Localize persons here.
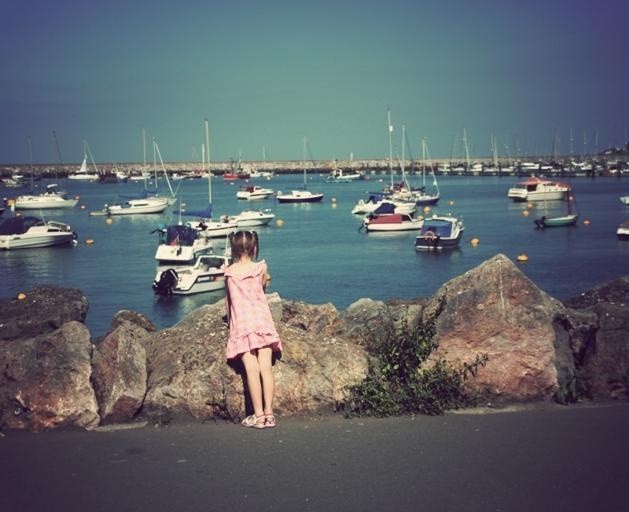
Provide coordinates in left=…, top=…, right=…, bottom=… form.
left=221, top=230, right=284, bottom=428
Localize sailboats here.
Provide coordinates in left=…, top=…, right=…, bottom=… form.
left=2, top=118, right=178, bottom=216
left=328, top=107, right=440, bottom=204
left=150, top=116, right=324, bottom=243
left=438, top=128, right=628, bottom=177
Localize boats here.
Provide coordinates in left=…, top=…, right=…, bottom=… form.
left=352, top=194, right=466, bottom=250
left=0, top=220, right=77, bottom=250
left=154, top=225, right=235, bottom=296
left=535, top=213, right=580, bottom=227
left=616, top=220, right=629, bottom=236
left=507, top=174, right=571, bottom=202
left=620, top=196, right=629, bottom=204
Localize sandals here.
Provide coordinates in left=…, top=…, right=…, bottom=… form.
left=241, top=414, right=276, bottom=429
